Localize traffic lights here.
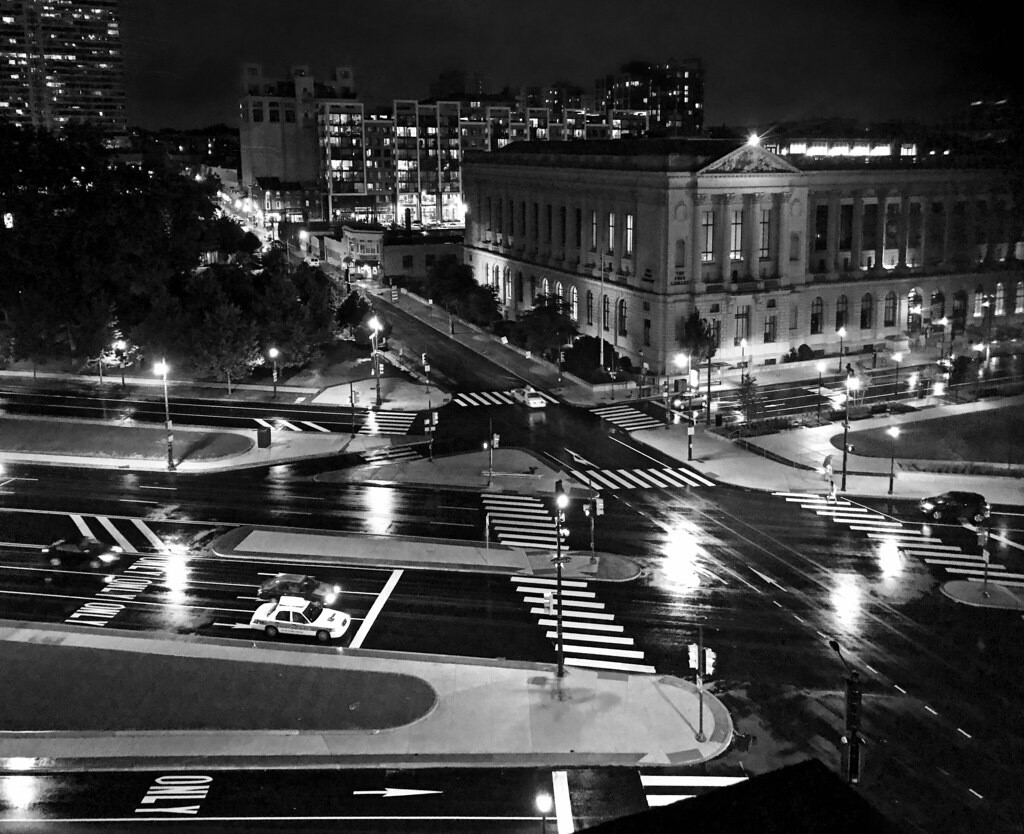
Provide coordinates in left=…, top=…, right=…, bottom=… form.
left=845, top=443, right=854, bottom=453
left=704, top=648, right=718, bottom=676
left=687, top=643, right=700, bottom=672
left=592, top=496, right=605, bottom=518
left=493, top=433, right=500, bottom=448
left=560, top=351, right=565, bottom=363
left=432, top=411, right=438, bottom=428
left=422, top=353, right=427, bottom=367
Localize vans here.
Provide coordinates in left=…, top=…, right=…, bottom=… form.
left=303, top=255, right=320, bottom=267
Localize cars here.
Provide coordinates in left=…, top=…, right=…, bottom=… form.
left=668, top=389, right=708, bottom=410
left=368, top=315, right=384, bottom=405
left=41, top=536, right=125, bottom=570
left=249, top=595, right=351, bottom=644
left=510, top=384, right=547, bottom=408
left=257, top=573, right=343, bottom=609
left=917, top=491, right=991, bottom=524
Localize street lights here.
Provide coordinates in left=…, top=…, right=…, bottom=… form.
left=841, top=361, right=860, bottom=492
left=549, top=480, right=571, bottom=678
left=740, top=338, right=748, bottom=385
left=827, top=639, right=862, bottom=783
left=429, top=299, right=433, bottom=328
left=269, top=347, right=279, bottom=398
left=116, top=341, right=127, bottom=387
left=817, top=361, right=827, bottom=423
left=891, top=351, right=903, bottom=401
left=886, top=426, right=901, bottom=494
left=836, top=326, right=847, bottom=374
left=152, top=355, right=177, bottom=471
left=939, top=316, right=948, bottom=360
